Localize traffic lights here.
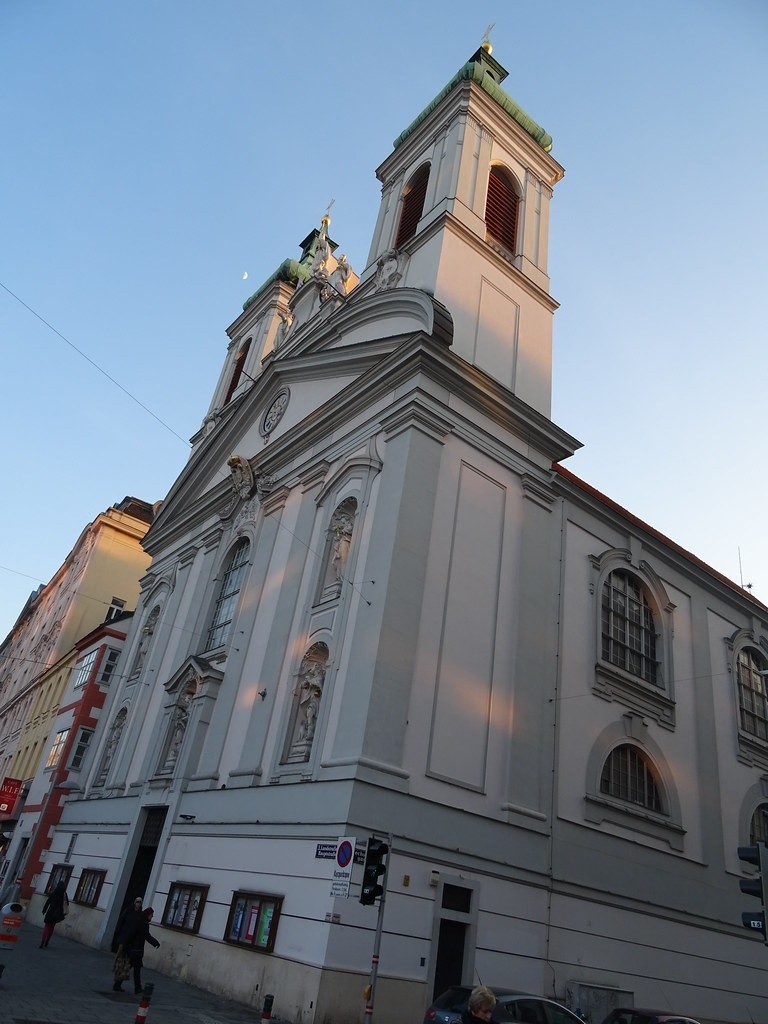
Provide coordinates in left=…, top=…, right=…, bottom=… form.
left=359, top=837, right=389, bottom=906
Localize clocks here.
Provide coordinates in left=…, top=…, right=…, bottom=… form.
left=258, top=386, right=290, bottom=437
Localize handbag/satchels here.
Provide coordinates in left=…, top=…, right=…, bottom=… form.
left=114, top=952, right=131, bottom=981
left=63, top=901, right=69, bottom=915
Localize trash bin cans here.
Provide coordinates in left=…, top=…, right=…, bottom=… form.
left=0, top=902, right=27, bottom=951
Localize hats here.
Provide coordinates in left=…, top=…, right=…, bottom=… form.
left=134, top=897, right=142, bottom=904
left=144, top=907, right=154, bottom=917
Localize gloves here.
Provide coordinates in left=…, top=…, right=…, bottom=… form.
left=155, top=943, right=160, bottom=950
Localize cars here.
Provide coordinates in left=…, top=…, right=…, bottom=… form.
left=423, top=983, right=589, bottom=1024
left=600, top=1007, right=702, bottom=1024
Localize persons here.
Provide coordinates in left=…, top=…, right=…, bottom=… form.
left=39, top=881, right=69, bottom=949
left=462, top=987, right=495, bottom=1024
left=331, top=514, right=353, bottom=579
left=296, top=663, right=322, bottom=741
left=171, top=695, right=192, bottom=749
left=327, top=255, right=352, bottom=295
left=137, top=618, right=156, bottom=669
left=113, top=897, right=143, bottom=971
left=113, top=907, right=160, bottom=994
left=274, top=309, right=289, bottom=347
left=111, top=718, right=126, bottom=749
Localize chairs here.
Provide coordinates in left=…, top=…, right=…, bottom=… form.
left=521, top=1008, right=541, bottom=1024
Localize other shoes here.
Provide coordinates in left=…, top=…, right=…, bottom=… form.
left=44, top=942, right=48, bottom=948
left=113, top=986, right=125, bottom=993
left=39, top=943, right=44, bottom=949
left=135, top=989, right=143, bottom=994
